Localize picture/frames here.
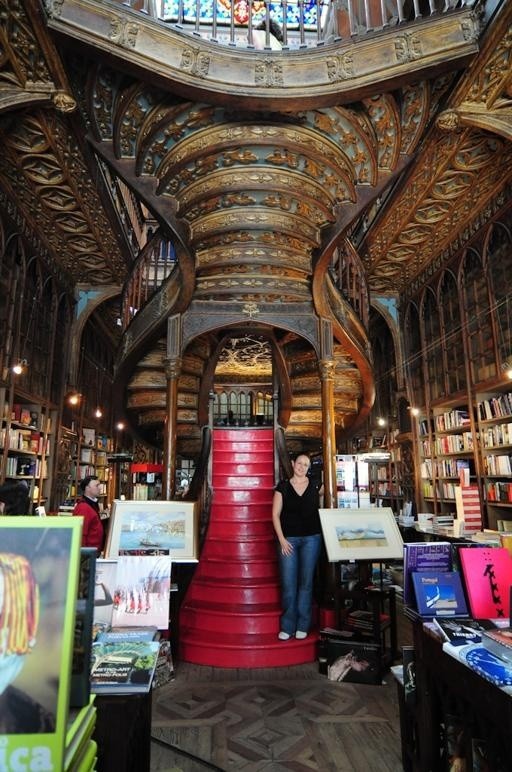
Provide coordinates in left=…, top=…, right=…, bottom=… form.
left=317, top=507, right=406, bottom=564
left=101, top=498, right=201, bottom=566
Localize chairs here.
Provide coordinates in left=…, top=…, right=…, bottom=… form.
left=315, top=587, right=397, bottom=685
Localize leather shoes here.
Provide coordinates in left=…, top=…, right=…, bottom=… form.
left=278, top=631, right=307, bottom=640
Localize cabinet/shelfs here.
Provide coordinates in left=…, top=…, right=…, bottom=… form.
left=348, top=393, right=483, bottom=532
left=387, top=658, right=420, bottom=772
left=94, top=684, right=154, bottom=772
left=0, top=378, right=56, bottom=524
left=470, top=374, right=511, bottom=534
left=58, top=415, right=117, bottom=518
left=399, top=603, right=512, bottom=772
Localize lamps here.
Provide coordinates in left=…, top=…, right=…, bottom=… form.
left=10, top=357, right=34, bottom=376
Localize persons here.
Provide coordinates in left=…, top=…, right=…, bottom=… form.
left=270, top=452, right=325, bottom=644
left=246, top=17, right=289, bottom=52
left=72, top=475, right=105, bottom=556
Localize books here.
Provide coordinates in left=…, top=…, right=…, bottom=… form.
left=335, top=427, right=401, bottom=508
left=132, top=472, right=164, bottom=500
left=68, top=426, right=114, bottom=511
left=1, top=502, right=174, bottom=770
left=400, top=389, right=512, bottom=697
left=2, top=400, right=52, bottom=511
